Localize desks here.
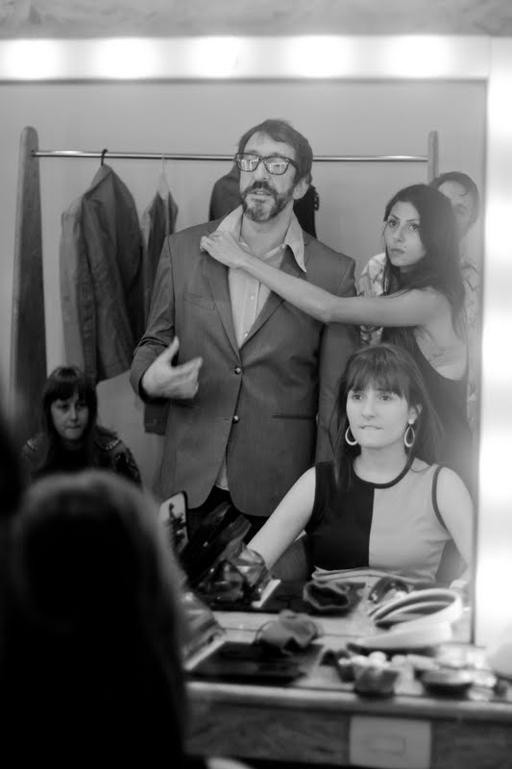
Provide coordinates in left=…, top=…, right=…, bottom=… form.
left=188, top=655, right=508, bottom=768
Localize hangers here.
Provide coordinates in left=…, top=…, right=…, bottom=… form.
left=60, top=147, right=135, bottom=222
left=144, top=151, right=179, bottom=221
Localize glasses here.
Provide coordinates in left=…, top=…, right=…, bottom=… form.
left=236, top=152, right=300, bottom=176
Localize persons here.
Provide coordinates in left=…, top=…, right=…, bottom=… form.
left=357, top=171, right=482, bottom=469
left=231, top=346, right=475, bottom=589
left=130, top=118, right=357, bottom=576
left=19, top=365, right=143, bottom=488
left=1, top=470, right=246, bottom=769
left=200, top=183, right=472, bottom=588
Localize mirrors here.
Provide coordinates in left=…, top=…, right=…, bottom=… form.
left=1, top=32, right=505, bottom=687
